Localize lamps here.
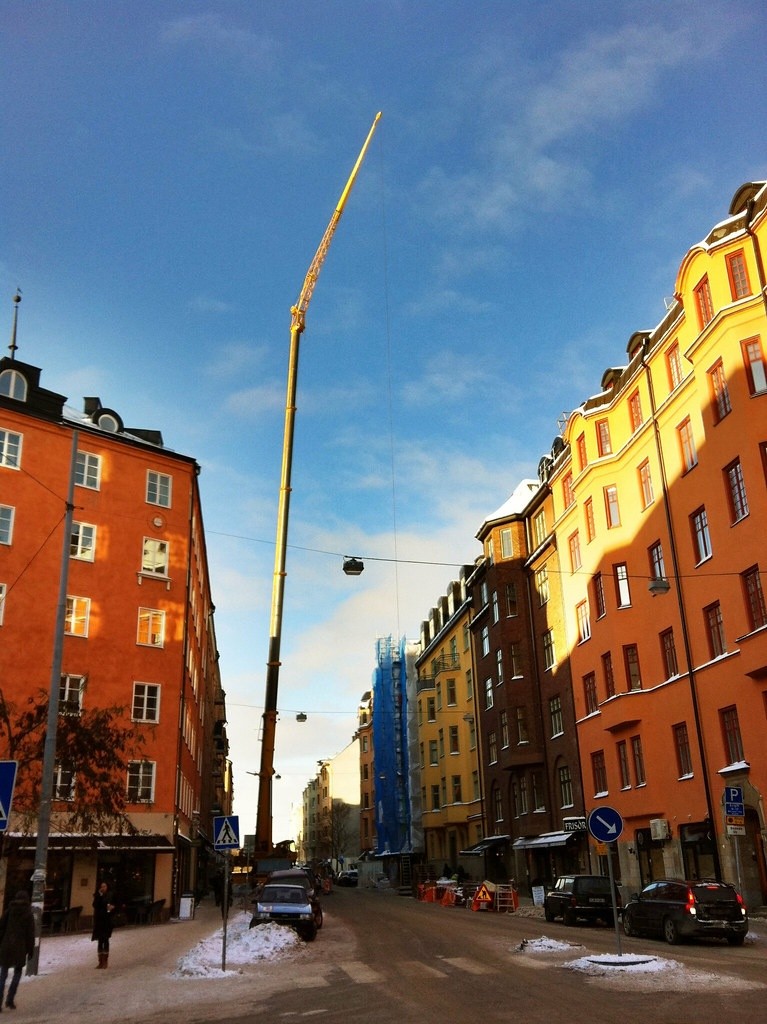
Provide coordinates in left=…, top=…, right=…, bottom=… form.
left=379, top=774, right=387, bottom=779
left=296, top=712, right=307, bottom=723
left=341, top=556, right=364, bottom=574
left=274, top=774, right=281, bottom=779
left=462, top=713, right=475, bottom=723
left=648, top=577, right=671, bottom=598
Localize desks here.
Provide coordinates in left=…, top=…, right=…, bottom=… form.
left=42, top=910, right=63, bottom=933
left=112, top=899, right=145, bottom=926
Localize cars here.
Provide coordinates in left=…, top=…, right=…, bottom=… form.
left=250, top=886, right=316, bottom=941
left=334, top=872, right=358, bottom=885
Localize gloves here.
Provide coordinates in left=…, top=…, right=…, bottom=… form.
left=28, top=950, right=33, bottom=960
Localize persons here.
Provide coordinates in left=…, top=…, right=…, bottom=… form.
left=215, top=869, right=233, bottom=918
left=444, top=862, right=452, bottom=879
left=0, top=891, right=35, bottom=1012
left=91, top=881, right=117, bottom=969
left=315, top=872, right=322, bottom=895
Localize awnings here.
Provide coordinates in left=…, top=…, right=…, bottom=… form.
left=458, top=834, right=509, bottom=856
left=512, top=831, right=574, bottom=850
left=19, top=836, right=174, bottom=850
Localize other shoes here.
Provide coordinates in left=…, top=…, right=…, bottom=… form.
left=5, top=999, right=16, bottom=1009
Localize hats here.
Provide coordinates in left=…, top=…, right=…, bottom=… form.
left=328, top=858, right=331, bottom=862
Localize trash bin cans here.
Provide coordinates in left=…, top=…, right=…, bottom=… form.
left=178, top=894, right=195, bottom=920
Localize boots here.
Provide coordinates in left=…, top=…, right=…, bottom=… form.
left=94, top=954, right=102, bottom=969
left=101, top=953, right=108, bottom=969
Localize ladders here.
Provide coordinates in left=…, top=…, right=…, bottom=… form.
left=494, top=884, right=516, bottom=912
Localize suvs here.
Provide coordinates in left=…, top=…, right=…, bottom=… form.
left=263, top=869, right=324, bottom=929
left=545, top=874, right=623, bottom=926
left=622, top=878, right=749, bottom=946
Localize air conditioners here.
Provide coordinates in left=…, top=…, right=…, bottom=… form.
left=179, top=898, right=194, bottom=921
left=650, top=819, right=669, bottom=841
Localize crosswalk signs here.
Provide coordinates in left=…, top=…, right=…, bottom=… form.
left=213, top=816, right=240, bottom=849
left=0, top=761, right=20, bottom=833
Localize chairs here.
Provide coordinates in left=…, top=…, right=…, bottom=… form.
left=290, top=892, right=301, bottom=902
left=51, top=906, right=83, bottom=934
left=265, top=892, right=278, bottom=902
left=136, top=899, right=167, bottom=926
